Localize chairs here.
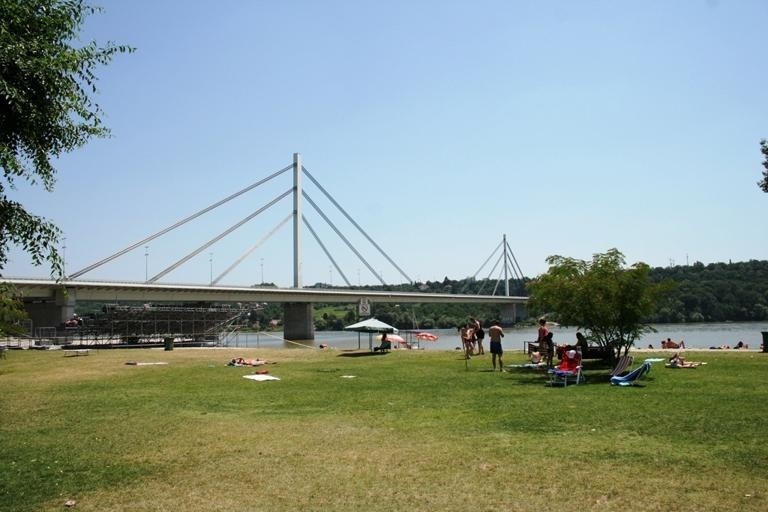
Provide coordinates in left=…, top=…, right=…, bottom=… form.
left=609, top=361, right=651, bottom=386
left=608, top=355, right=632, bottom=377
left=546, top=352, right=586, bottom=389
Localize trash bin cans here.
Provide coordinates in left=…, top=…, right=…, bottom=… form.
left=761, top=331, right=768, bottom=353
left=164, top=337, right=174, bottom=351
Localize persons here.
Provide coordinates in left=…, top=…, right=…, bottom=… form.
left=568, top=332, right=589, bottom=359
left=530, top=318, right=557, bottom=369
left=488, top=319, right=504, bottom=372
left=661, top=341, right=667, bottom=349
left=666, top=338, right=685, bottom=349
left=382, top=333, right=387, bottom=352
left=461, top=317, right=485, bottom=360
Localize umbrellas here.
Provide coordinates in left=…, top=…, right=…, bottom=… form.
left=343, top=317, right=400, bottom=353
